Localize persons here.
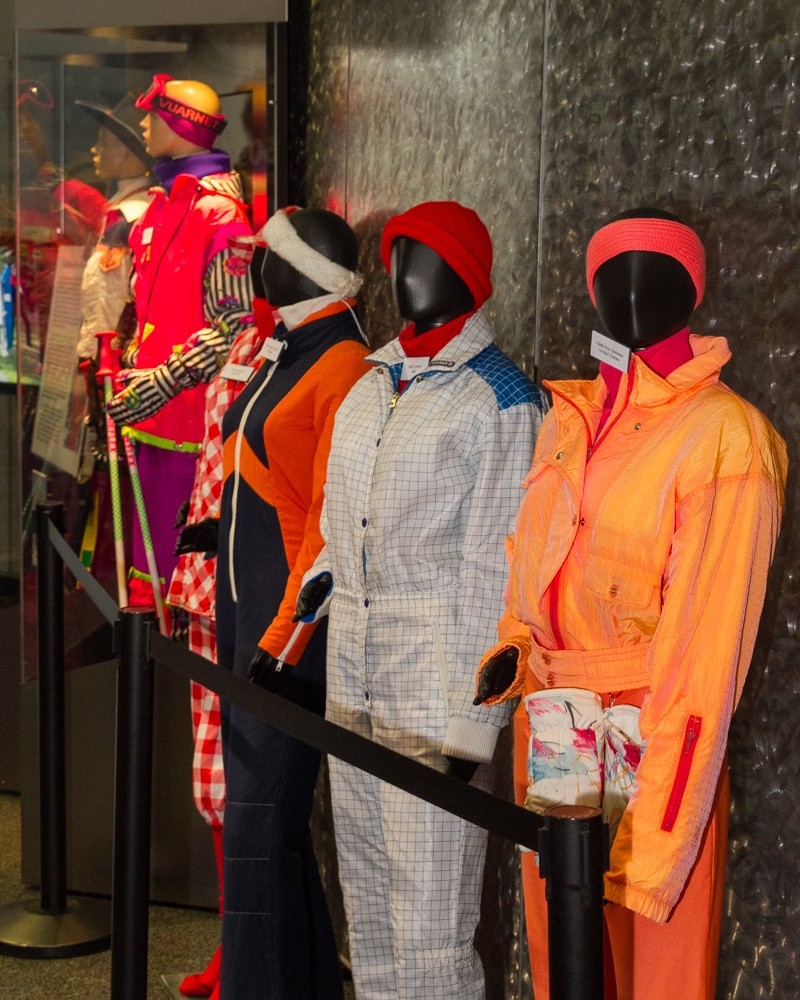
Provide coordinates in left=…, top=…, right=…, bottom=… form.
left=1, top=65, right=372, bottom=1000
left=498, top=201, right=790, bottom=1000
left=292, top=198, right=589, bottom=1000
